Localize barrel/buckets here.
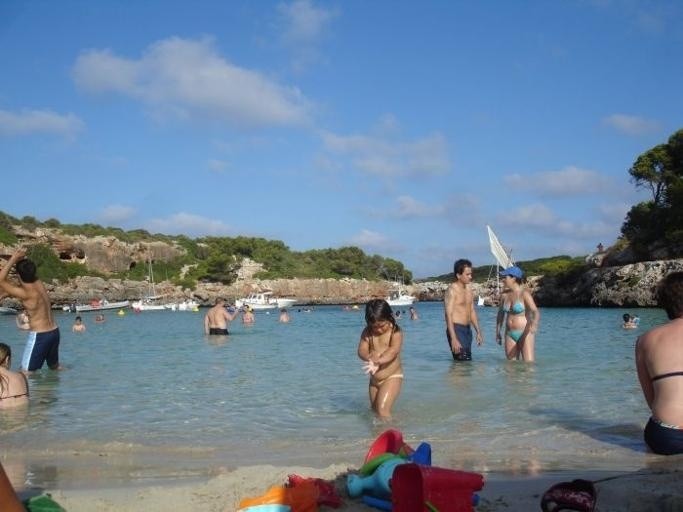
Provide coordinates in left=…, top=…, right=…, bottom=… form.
left=363, top=428, right=416, bottom=465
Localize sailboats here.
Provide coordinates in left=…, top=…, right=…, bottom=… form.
left=132, top=248, right=199, bottom=312
left=479, top=225, right=544, bottom=308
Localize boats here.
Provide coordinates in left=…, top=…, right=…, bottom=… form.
left=0, top=305, right=16, bottom=315
left=383, top=271, right=414, bottom=307
left=51, top=304, right=62, bottom=310
left=234, top=289, right=298, bottom=313
left=63, top=293, right=130, bottom=313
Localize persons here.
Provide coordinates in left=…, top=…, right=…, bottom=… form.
left=445, top=259, right=484, bottom=361
left=636, top=271, right=682, bottom=455
left=597, top=243, right=604, bottom=252
left=358, top=299, right=404, bottom=417
left=395, top=307, right=420, bottom=321
left=72, top=307, right=141, bottom=333
left=279, top=305, right=316, bottom=322
left=342, top=302, right=361, bottom=312
left=621, top=314, right=638, bottom=328
left=193, top=297, right=258, bottom=336
left=0, top=461, right=28, bottom=512
left=0, top=244, right=62, bottom=373
left=496, top=267, right=539, bottom=362
left=0, top=343, right=30, bottom=405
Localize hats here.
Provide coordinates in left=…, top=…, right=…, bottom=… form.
left=498, top=266, right=522, bottom=279
